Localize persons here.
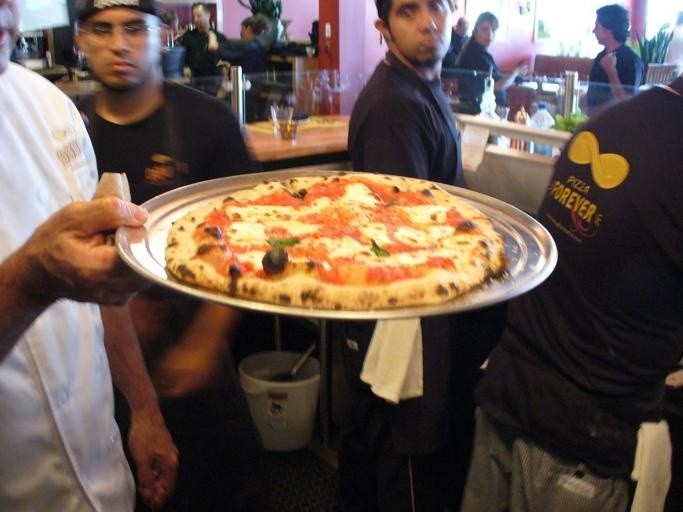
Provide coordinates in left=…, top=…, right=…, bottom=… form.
left=582, top=5, right=646, bottom=108
left=438, top=13, right=528, bottom=114
left=182, top=1, right=271, bottom=122
left=1, top=0, right=180, bottom=512
left=64, top=0, right=264, bottom=511
left=459, top=73, right=682, bottom=511
left=334, top=0, right=471, bottom=511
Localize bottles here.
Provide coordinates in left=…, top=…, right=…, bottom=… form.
left=526, top=101, right=556, bottom=158
left=474, top=77, right=502, bottom=148
left=508, top=105, right=531, bottom=153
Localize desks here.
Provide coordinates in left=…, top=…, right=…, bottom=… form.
left=512, top=74, right=585, bottom=102
left=247, top=112, right=350, bottom=163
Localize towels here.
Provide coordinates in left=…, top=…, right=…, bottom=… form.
left=360, top=318, right=429, bottom=405
left=627, top=419, right=670, bottom=511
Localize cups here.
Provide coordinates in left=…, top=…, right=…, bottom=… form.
left=279, top=120, right=297, bottom=140
left=270, top=105, right=293, bottom=136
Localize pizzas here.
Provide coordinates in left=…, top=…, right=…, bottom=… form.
left=165, top=171, right=506, bottom=311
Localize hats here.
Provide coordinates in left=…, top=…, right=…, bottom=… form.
left=75, top=1, right=165, bottom=29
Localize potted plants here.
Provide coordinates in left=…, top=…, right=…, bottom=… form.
left=632, top=21, right=682, bottom=84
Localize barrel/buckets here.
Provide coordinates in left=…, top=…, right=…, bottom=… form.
left=237, top=351, right=321, bottom=451
